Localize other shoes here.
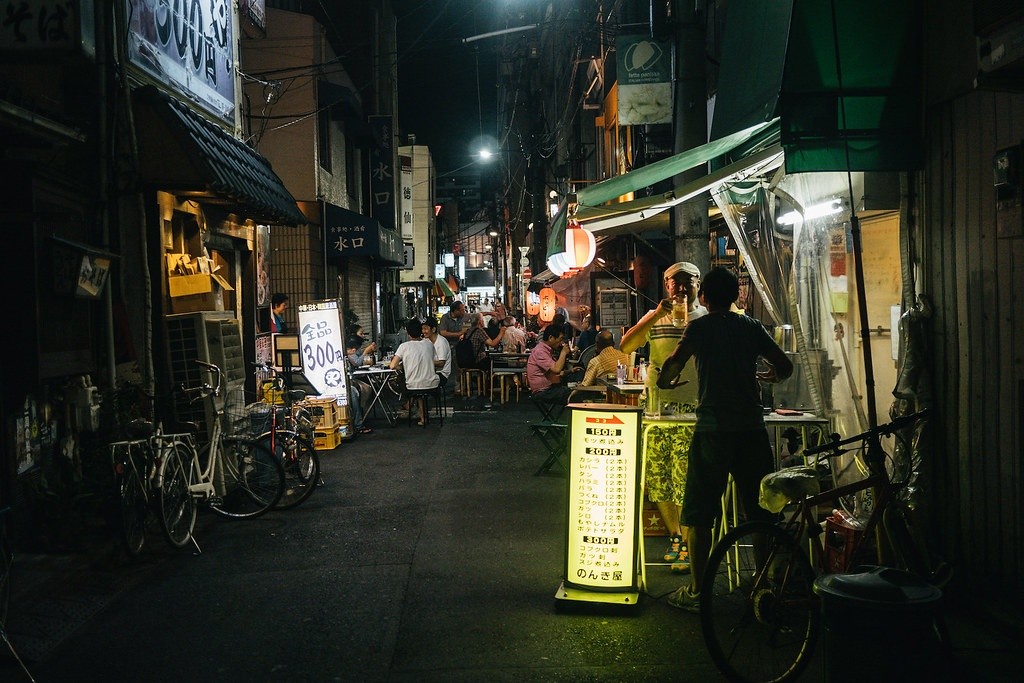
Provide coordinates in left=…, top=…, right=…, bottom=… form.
left=418, top=419, right=431, bottom=426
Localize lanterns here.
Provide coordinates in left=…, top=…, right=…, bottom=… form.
left=526, top=290, right=539, bottom=316
left=547, top=225, right=596, bottom=279
left=539, top=285, right=556, bottom=323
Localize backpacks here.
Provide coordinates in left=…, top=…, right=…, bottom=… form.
left=456, top=327, right=484, bottom=368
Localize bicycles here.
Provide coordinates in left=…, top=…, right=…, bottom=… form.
left=150, top=360, right=285, bottom=556
left=699, top=391, right=955, bottom=683
left=238, top=361, right=321, bottom=513
left=107, top=379, right=169, bottom=557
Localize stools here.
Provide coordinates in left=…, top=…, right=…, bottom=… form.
left=407, top=363, right=570, bottom=476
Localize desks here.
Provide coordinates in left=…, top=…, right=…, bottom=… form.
left=353, top=370, right=398, bottom=428
left=358, top=359, right=406, bottom=416
left=487, top=352, right=559, bottom=401
left=631, top=409, right=833, bottom=592
left=596, top=376, right=645, bottom=406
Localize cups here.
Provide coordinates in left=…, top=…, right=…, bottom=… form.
left=387, top=351, right=394, bottom=360
left=666, top=294, right=688, bottom=329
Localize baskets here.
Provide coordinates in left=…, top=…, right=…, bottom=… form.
left=223, top=388, right=272, bottom=443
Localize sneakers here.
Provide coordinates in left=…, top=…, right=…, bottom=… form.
left=666, top=584, right=702, bottom=614
left=663, top=534, right=683, bottom=562
left=670, top=542, right=691, bottom=572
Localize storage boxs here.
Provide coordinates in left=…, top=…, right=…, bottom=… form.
left=167, top=273, right=235, bottom=312
left=261, top=383, right=348, bottom=450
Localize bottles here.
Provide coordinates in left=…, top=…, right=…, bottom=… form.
left=617, top=365, right=623, bottom=385
left=516, top=341, right=521, bottom=353
left=639, top=357, right=647, bottom=381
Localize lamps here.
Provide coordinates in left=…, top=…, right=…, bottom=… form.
left=776, top=196, right=851, bottom=225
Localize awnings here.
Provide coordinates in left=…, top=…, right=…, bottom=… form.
left=321, top=201, right=404, bottom=266
left=777, top=0, right=1024, bottom=173
left=531, top=268, right=557, bottom=283
left=133, top=86, right=307, bottom=227
left=545, top=116, right=784, bottom=254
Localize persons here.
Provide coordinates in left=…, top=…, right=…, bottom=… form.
left=659, top=265, right=793, bottom=615
left=618, top=261, right=709, bottom=572
left=271, top=293, right=288, bottom=333
left=343, top=297, right=632, bottom=433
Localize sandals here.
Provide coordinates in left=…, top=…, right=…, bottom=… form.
left=358, top=428, right=372, bottom=435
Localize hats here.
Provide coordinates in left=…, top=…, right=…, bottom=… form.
left=664, top=262, right=701, bottom=280
left=421, top=317, right=438, bottom=327
